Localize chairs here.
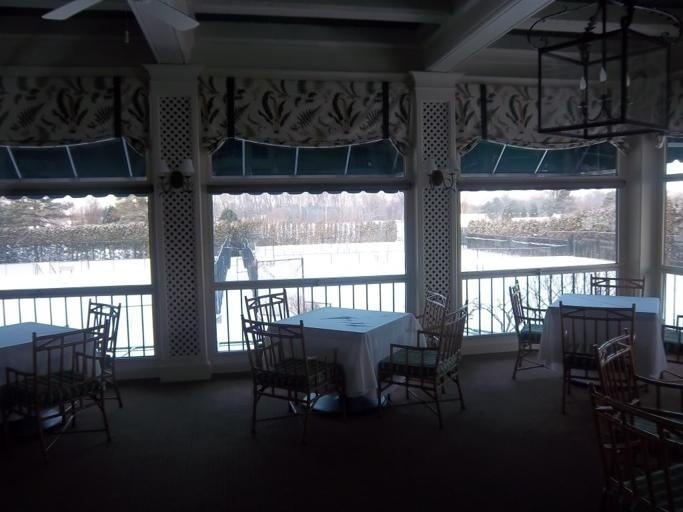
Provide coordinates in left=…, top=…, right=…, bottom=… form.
left=659, top=315, right=682, bottom=382
left=559, top=300, right=636, bottom=416
left=240, top=287, right=468, bottom=445
left=590, top=275, right=644, bottom=298
left=589, top=328, right=682, bottom=510
left=0, top=298, right=123, bottom=464
left=509, top=280, right=547, bottom=379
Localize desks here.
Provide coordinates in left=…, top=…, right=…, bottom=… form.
left=547, top=293, right=660, bottom=395
